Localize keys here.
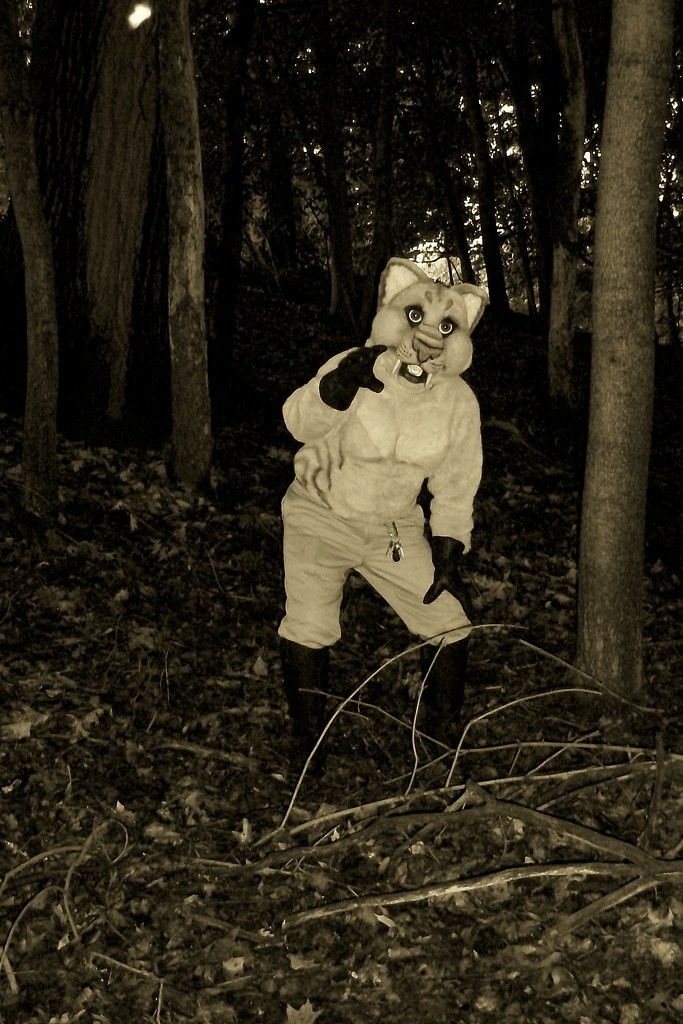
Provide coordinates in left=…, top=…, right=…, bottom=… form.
left=385, top=540, right=406, bottom=562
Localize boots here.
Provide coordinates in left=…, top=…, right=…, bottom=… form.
left=278, top=636, right=331, bottom=791
left=418, top=638, right=465, bottom=770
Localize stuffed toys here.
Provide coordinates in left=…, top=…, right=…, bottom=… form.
left=280, top=254, right=491, bottom=791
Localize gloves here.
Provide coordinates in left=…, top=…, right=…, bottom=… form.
left=422, top=537, right=475, bottom=622
left=319, top=345, right=383, bottom=412
